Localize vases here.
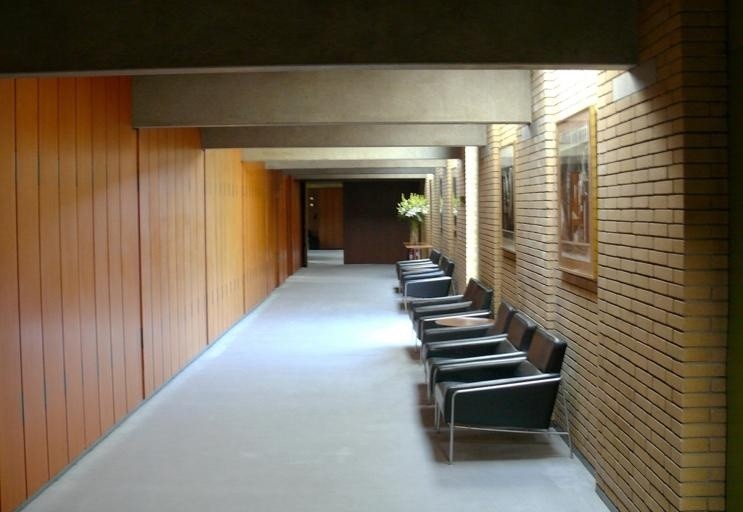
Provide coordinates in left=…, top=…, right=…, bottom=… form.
left=409, top=220, right=419, bottom=245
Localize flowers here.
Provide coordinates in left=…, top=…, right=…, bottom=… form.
left=394, top=191, right=429, bottom=226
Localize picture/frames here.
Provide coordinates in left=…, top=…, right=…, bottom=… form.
left=499, top=140, right=516, bottom=255
left=451, top=166, right=459, bottom=233
left=556, top=105, right=597, bottom=281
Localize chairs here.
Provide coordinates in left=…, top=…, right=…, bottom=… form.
left=396, top=250, right=455, bottom=312
left=409, top=278, right=573, bottom=465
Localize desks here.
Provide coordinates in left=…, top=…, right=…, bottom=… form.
left=402, top=241, right=433, bottom=260
